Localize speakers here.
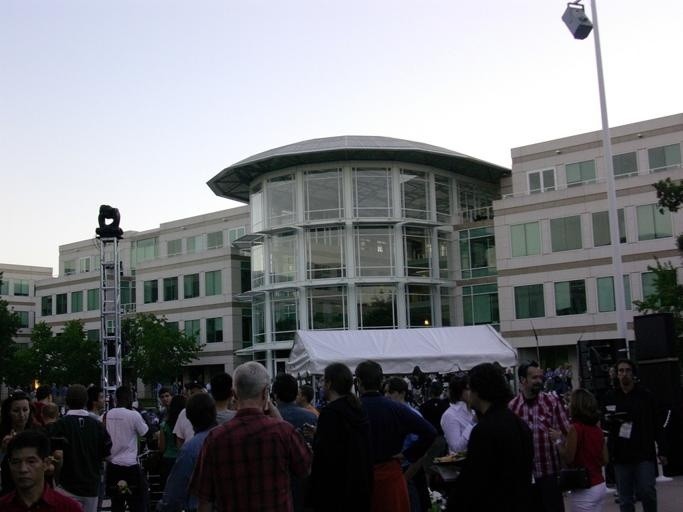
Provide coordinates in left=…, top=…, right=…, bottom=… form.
left=638, top=358, right=682, bottom=477
left=633, top=312, right=675, bottom=361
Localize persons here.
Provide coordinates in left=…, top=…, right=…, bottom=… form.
left=607, top=366, right=616, bottom=387
left=0, top=358, right=609, bottom=512
left=600, top=359, right=671, bottom=512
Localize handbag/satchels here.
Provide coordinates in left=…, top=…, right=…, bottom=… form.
left=560, top=465, right=591, bottom=489
